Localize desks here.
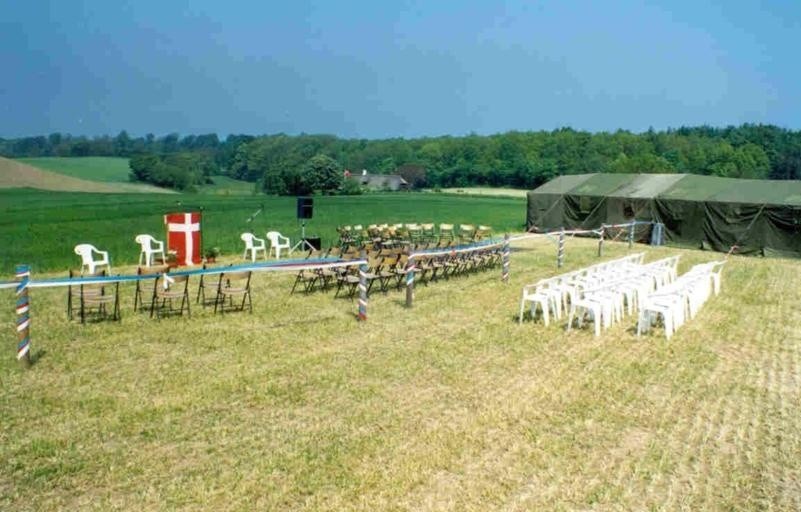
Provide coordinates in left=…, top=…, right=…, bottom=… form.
left=60, top=257, right=367, bottom=287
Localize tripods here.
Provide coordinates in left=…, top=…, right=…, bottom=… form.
left=287, top=224, right=318, bottom=255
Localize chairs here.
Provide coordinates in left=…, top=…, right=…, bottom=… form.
left=134, top=267, right=172, bottom=313
left=67, top=270, right=107, bottom=322
left=76, top=244, right=111, bottom=277
left=149, top=275, right=191, bottom=321
left=213, top=270, right=254, bottom=317
left=135, top=235, right=166, bottom=268
left=266, top=232, right=290, bottom=260
left=290, top=221, right=508, bottom=306
left=519, top=249, right=730, bottom=341
left=79, top=274, right=121, bottom=327
left=196, top=263, right=233, bottom=308
left=241, top=233, right=266, bottom=262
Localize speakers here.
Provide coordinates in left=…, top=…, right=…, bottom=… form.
left=297, top=198, right=313, bottom=220
left=305, top=237, right=321, bottom=251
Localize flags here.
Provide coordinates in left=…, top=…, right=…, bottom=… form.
left=165, top=212, right=202, bottom=266
